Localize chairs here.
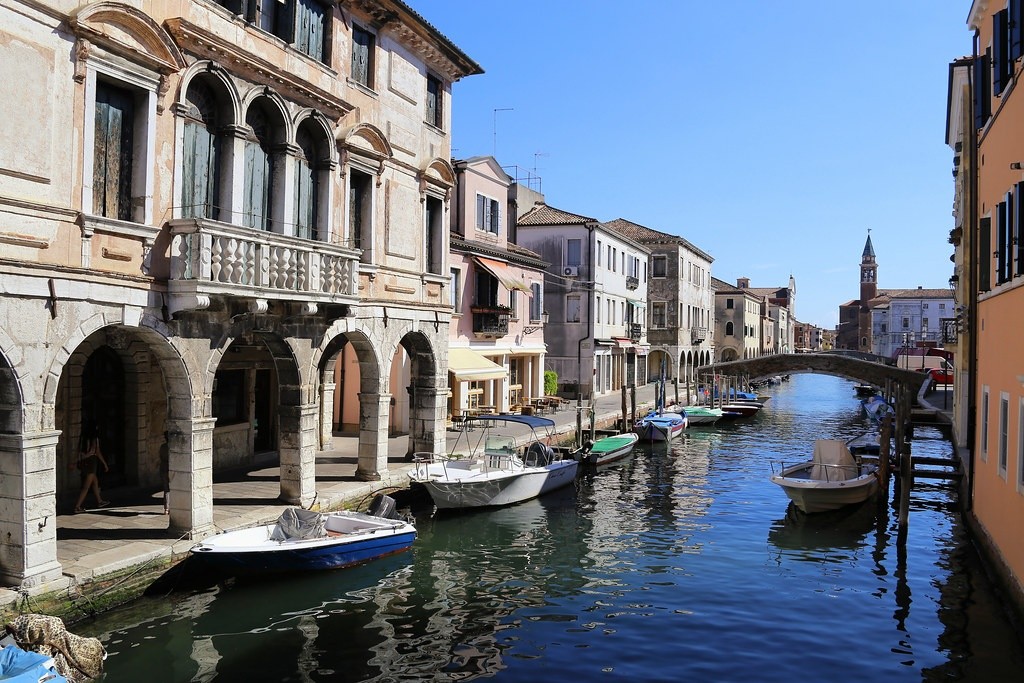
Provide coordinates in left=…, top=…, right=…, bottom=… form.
left=448, top=393, right=574, bottom=434
left=520, top=450, right=539, bottom=465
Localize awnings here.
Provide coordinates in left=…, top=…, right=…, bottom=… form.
left=626, top=299, right=645, bottom=307
left=472, top=256, right=534, bottom=298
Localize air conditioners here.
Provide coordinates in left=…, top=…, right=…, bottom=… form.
left=564, top=267, right=578, bottom=276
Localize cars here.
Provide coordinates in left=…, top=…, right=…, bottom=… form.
left=926, top=368, right=955, bottom=385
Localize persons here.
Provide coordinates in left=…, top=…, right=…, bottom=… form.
left=159, top=430, right=170, bottom=515
left=71, top=416, right=111, bottom=514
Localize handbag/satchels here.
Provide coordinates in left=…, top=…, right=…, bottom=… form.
left=271, top=508, right=329, bottom=540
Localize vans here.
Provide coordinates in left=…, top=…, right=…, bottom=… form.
left=897, top=354, right=954, bottom=373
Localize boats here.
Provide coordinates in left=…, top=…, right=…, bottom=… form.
left=680, top=403, right=724, bottom=426
left=407, top=412, right=573, bottom=514
left=187, top=484, right=418, bottom=585
left=564, top=431, right=639, bottom=466
left=632, top=403, right=690, bottom=442
left=770, top=439, right=880, bottom=516
left=699, top=371, right=790, bottom=420
